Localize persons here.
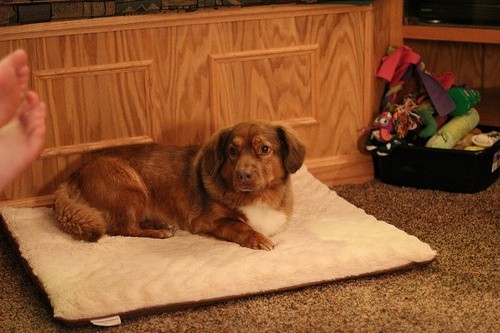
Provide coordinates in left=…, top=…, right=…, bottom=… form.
left=0, top=49, right=47, bottom=196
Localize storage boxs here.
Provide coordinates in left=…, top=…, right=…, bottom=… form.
left=367, top=123, right=500, bottom=195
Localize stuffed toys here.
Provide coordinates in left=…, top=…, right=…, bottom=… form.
left=365, top=111, right=395, bottom=157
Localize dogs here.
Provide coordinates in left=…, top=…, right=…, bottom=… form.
left=53, top=120, right=305, bottom=251
